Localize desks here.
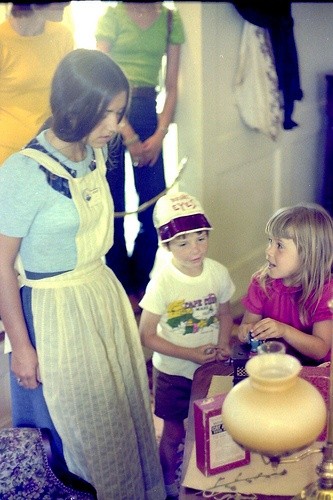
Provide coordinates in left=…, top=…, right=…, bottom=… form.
left=179, top=361, right=333, bottom=500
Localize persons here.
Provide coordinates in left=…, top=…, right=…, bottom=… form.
left=239, top=205, right=333, bottom=370
left=98, top=1, right=184, bottom=304
left=1, top=1, right=76, bottom=163
left=138, top=191, right=235, bottom=500
left=0, top=47, right=168, bottom=500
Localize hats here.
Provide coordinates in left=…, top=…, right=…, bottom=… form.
left=153, top=192, right=212, bottom=243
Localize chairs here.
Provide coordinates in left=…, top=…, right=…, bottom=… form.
left=0, top=427, right=98, bottom=500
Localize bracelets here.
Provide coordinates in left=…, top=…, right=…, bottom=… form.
left=124, top=134, right=139, bottom=144
left=158, top=128, right=168, bottom=135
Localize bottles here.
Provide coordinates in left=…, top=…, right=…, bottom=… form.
left=221, top=354, right=327, bottom=457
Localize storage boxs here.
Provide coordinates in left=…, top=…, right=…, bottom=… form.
left=192, top=393, right=250, bottom=477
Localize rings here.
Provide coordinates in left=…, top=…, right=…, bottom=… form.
left=17, top=379, right=21, bottom=383
left=134, top=162, right=139, bottom=167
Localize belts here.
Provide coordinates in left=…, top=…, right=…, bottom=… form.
left=131, top=87, right=154, bottom=98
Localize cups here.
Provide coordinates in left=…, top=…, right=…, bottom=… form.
left=256, top=341, right=285, bottom=355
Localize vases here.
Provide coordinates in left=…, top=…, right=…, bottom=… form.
left=223, top=354, right=326, bottom=456
